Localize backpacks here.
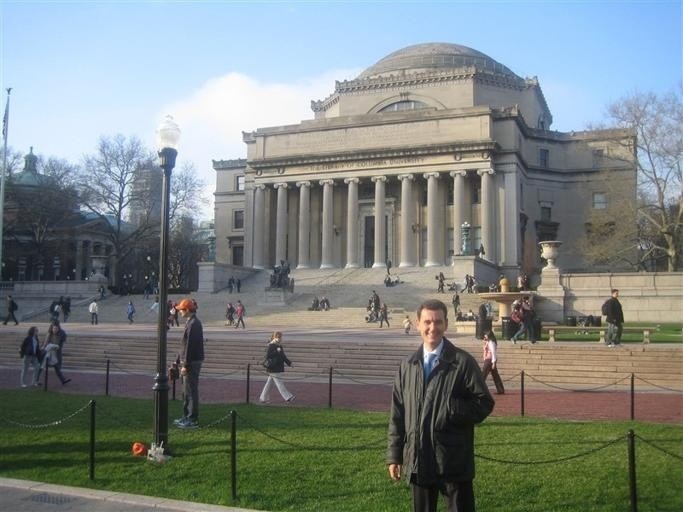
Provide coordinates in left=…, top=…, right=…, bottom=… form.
left=601, top=299, right=610, bottom=316
left=13, top=302, right=18, bottom=311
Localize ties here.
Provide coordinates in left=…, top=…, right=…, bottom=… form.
left=423, top=354, right=437, bottom=390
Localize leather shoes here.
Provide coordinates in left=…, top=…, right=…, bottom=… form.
left=494, top=391, right=504, bottom=395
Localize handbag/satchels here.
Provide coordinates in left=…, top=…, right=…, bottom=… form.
left=170, top=308, right=176, bottom=316
left=262, top=356, right=279, bottom=368
left=511, top=311, right=523, bottom=324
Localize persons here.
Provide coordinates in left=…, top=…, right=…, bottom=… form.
left=480, top=329, right=506, bottom=393
left=225, top=299, right=246, bottom=328
left=308, top=295, right=329, bottom=310
left=37, top=322, right=73, bottom=390
left=172, top=299, right=205, bottom=428
left=364, top=258, right=413, bottom=334
left=49, top=293, right=73, bottom=321
left=386, top=300, right=496, bottom=511
left=601, top=288, right=625, bottom=350
left=151, top=295, right=199, bottom=328
left=259, top=331, right=297, bottom=404
left=121, top=271, right=136, bottom=299
left=18, top=325, right=44, bottom=387
left=124, top=300, right=137, bottom=323
left=142, top=274, right=152, bottom=299
left=435, top=271, right=539, bottom=345
left=88, top=298, right=100, bottom=327
left=98, top=285, right=107, bottom=298
left=226, top=275, right=242, bottom=294
left=1, top=293, right=20, bottom=326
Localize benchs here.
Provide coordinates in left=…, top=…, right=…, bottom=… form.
left=541, top=325, right=660, bottom=343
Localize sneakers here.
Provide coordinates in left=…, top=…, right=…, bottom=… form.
left=19, top=382, right=43, bottom=389
left=286, top=395, right=297, bottom=405
left=2, top=322, right=7, bottom=326
left=511, top=337, right=517, bottom=345
left=61, top=378, right=71, bottom=385
left=259, top=399, right=273, bottom=405
left=13, top=323, right=18, bottom=326
left=530, top=342, right=539, bottom=345
left=173, top=417, right=199, bottom=429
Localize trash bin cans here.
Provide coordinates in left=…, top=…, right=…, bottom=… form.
left=566, top=316, right=575, bottom=327
left=475, top=319, right=491, bottom=340
left=502, top=317, right=516, bottom=341
left=590, top=316, right=601, bottom=327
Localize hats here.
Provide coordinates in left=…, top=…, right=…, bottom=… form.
left=175, top=298, right=196, bottom=310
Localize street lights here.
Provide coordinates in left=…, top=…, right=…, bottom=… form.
left=461, top=221, right=472, bottom=256
left=151, top=114, right=181, bottom=446
